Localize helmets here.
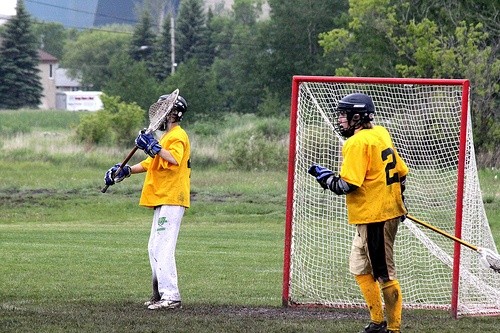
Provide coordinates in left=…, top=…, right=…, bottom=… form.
left=337, top=93, right=374, bottom=113
left=159, top=94, right=188, bottom=112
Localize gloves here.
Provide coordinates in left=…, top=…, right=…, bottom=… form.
left=308, top=165, right=332, bottom=185
left=104, top=163, right=132, bottom=186
left=134, top=129, right=161, bottom=159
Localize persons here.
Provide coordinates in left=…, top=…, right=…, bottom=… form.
left=104, top=94, right=191, bottom=311
left=308, top=93, right=409, bottom=333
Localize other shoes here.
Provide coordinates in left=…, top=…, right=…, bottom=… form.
left=145, top=300, right=183, bottom=311
left=363, top=320, right=401, bottom=333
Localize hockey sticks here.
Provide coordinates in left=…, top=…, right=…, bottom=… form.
left=101, top=88, right=180, bottom=193
left=406, top=213, right=500, bottom=274
